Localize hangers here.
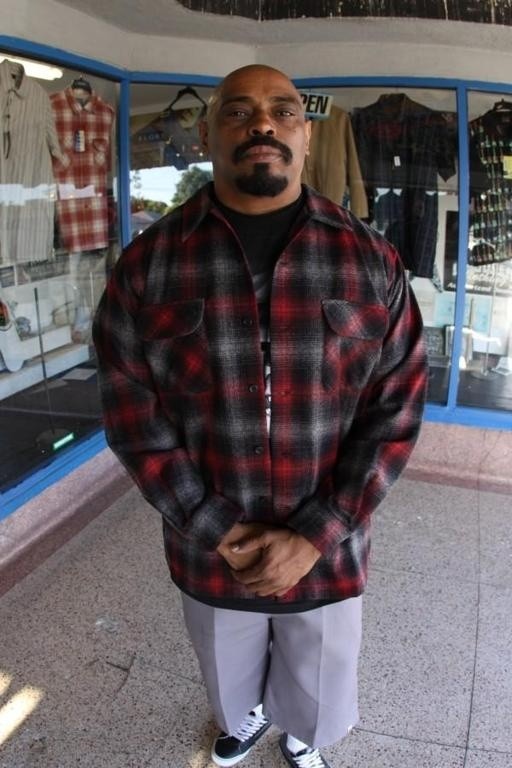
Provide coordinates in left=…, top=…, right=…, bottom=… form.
left=71, top=65, right=92, bottom=97
left=168, top=84, right=214, bottom=112
left=494, top=93, right=512, bottom=115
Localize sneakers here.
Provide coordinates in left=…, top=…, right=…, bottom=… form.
left=278, top=730, right=333, bottom=768
left=208, top=702, right=275, bottom=767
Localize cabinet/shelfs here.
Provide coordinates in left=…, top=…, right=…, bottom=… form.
left=1, top=36, right=219, bottom=522
left=290, top=77, right=511, bottom=432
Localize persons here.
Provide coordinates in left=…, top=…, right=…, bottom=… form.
left=91, top=64, right=431, bottom=768
